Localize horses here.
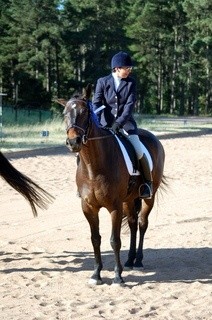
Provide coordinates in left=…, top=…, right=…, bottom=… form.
left=57, top=82, right=174, bottom=285
left=0, top=149, right=56, bottom=218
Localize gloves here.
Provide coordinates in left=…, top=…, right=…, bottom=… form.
left=111, top=122, right=119, bottom=135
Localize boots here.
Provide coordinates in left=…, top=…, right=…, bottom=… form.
left=137, top=153, right=154, bottom=198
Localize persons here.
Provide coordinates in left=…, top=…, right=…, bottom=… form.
left=92, top=51, right=154, bottom=199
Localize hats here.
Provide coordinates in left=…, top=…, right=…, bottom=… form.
left=111, top=51, right=138, bottom=67
